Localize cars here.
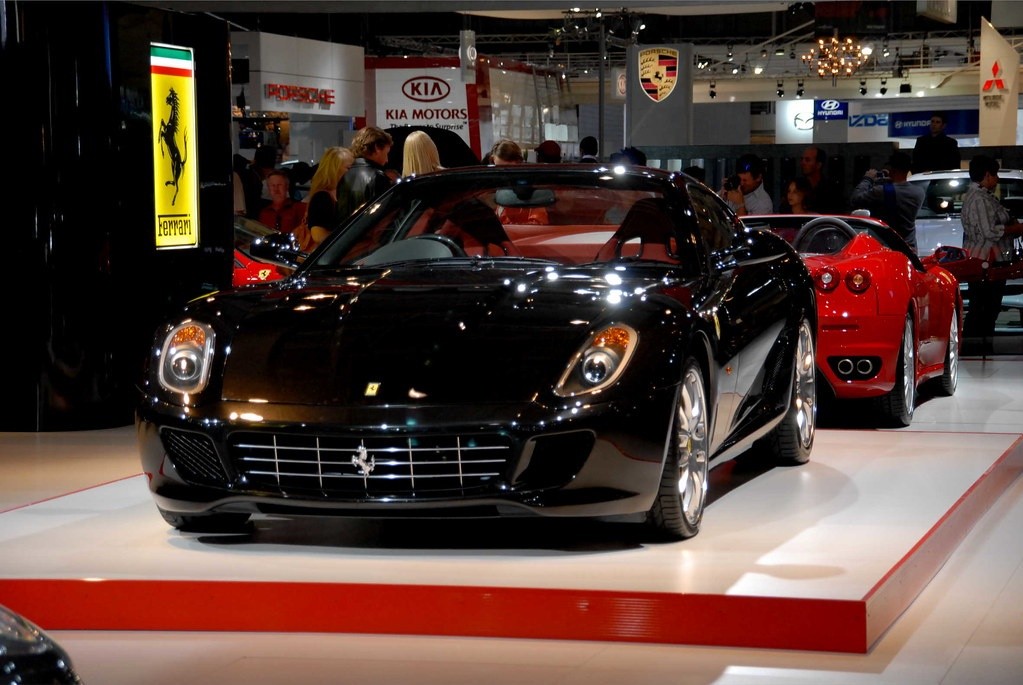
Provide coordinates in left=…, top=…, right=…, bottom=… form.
left=738, top=215, right=966, bottom=429
left=133, top=162, right=818, bottom=539
left=918, top=224, right=1023, bottom=355
left=231, top=213, right=303, bottom=287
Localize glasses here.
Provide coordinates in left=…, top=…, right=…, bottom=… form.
left=274, top=214, right=281, bottom=229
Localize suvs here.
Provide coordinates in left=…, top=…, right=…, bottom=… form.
left=854, top=167, right=1023, bottom=256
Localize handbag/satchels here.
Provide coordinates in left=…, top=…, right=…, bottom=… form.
left=988, top=258, right=1023, bottom=281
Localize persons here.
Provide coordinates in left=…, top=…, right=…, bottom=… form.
left=336, top=126, right=395, bottom=227
left=481, top=139, right=523, bottom=164
left=578, top=136, right=600, bottom=163
left=534, top=140, right=561, bottom=162
left=958, top=153, right=1023, bottom=356
left=850, top=151, right=925, bottom=256
left=401, top=130, right=447, bottom=178
left=783, top=146, right=839, bottom=214
left=715, top=153, right=773, bottom=214
left=293, top=146, right=354, bottom=253
left=259, top=171, right=306, bottom=232
left=911, top=113, right=962, bottom=175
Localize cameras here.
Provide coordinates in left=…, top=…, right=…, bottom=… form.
left=875, top=172, right=886, bottom=180
left=724, top=174, right=741, bottom=191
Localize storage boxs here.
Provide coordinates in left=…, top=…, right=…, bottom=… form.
left=591, top=198, right=683, bottom=265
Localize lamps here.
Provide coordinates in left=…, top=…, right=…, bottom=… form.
left=882, top=36, right=890, bottom=58
left=776, top=80, right=784, bottom=98
left=880, top=78, right=888, bottom=94
left=709, top=81, right=716, bottom=99
left=802, top=27, right=868, bottom=80
left=774, top=45, right=784, bottom=56
left=796, top=79, right=804, bottom=96
left=727, top=44, right=734, bottom=58
left=899, top=78, right=912, bottom=93
left=859, top=78, right=867, bottom=95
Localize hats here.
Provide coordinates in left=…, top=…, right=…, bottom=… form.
left=534, top=140, right=561, bottom=153
left=621, top=146, right=647, bottom=167
